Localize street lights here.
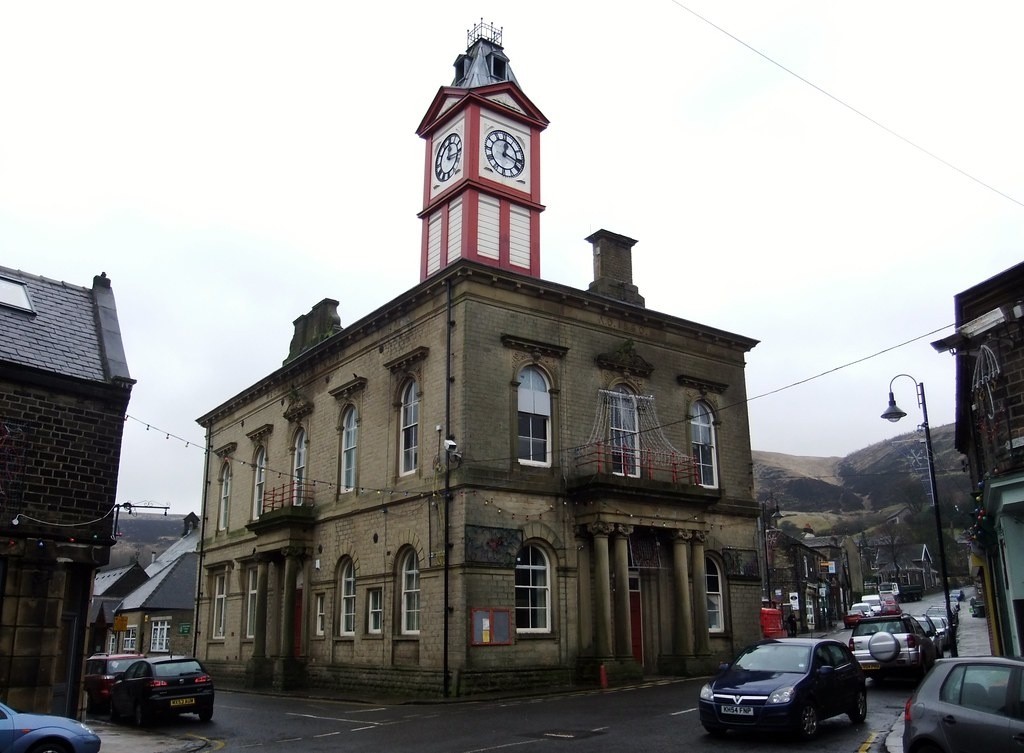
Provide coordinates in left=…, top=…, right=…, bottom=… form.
left=762, top=497, right=783, bottom=607
left=881, top=373, right=958, bottom=658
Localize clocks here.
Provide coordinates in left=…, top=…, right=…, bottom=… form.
left=485, top=130, right=525, bottom=178
left=434, top=133, right=462, bottom=182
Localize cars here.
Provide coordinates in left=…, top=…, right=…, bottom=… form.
left=698, top=638, right=867, bottom=741
left=949, top=590, right=965, bottom=601
left=913, top=598, right=961, bottom=657
left=902, top=656, right=1024, bottom=753
left=843, top=610, right=866, bottom=629
left=880, top=594, right=902, bottom=615
left=111, top=655, right=214, bottom=727
left=0, top=702, right=101, bottom=753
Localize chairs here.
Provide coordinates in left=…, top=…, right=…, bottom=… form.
left=866, top=625, right=879, bottom=635
left=886, top=624, right=898, bottom=634
left=959, top=683, right=1007, bottom=716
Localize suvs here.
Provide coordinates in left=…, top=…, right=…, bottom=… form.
left=83, top=653, right=146, bottom=713
left=849, top=613, right=939, bottom=685
left=851, top=603, right=875, bottom=618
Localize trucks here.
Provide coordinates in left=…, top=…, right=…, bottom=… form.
left=878, top=582, right=922, bottom=603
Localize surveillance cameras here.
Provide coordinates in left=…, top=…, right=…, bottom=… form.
left=443, top=439, right=457, bottom=452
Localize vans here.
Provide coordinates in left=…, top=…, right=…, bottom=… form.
left=861, top=594, right=881, bottom=615
left=762, top=599, right=792, bottom=637
